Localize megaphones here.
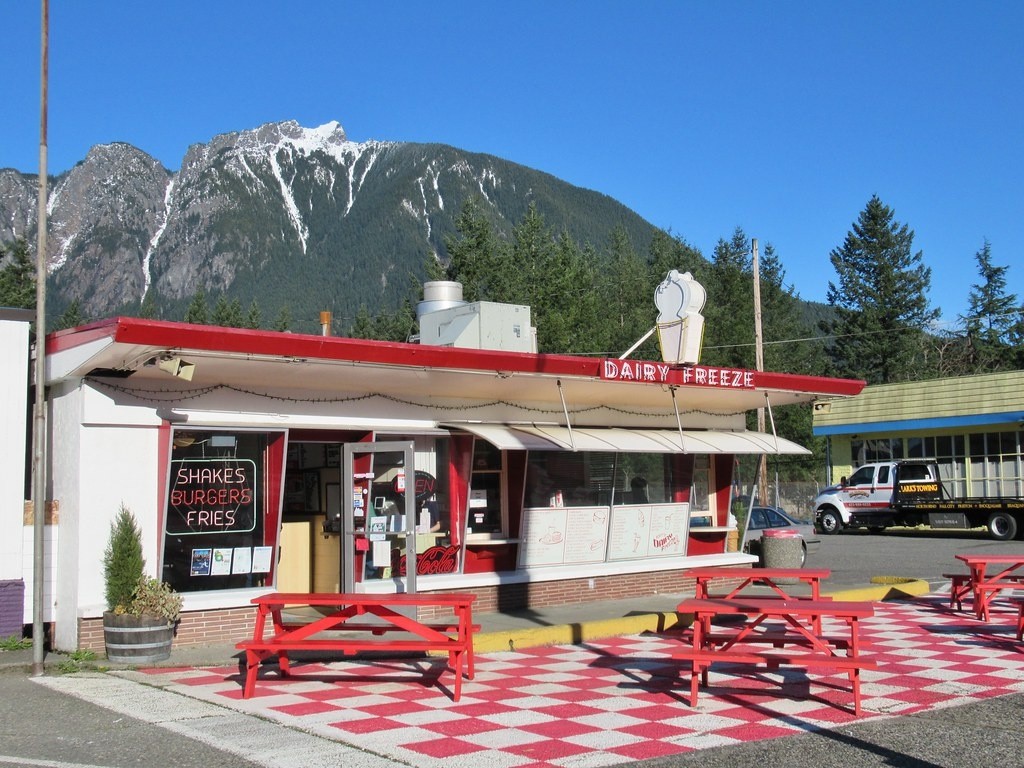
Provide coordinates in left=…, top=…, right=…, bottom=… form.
left=810, top=399, right=831, bottom=415
left=158, top=357, right=195, bottom=381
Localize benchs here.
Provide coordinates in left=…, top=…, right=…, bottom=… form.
left=277, top=622, right=481, bottom=680
left=942, top=574, right=1024, bottom=641
left=672, top=594, right=878, bottom=716
left=235, top=640, right=467, bottom=703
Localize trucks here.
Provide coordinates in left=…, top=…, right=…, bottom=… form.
left=813, top=458, right=1024, bottom=541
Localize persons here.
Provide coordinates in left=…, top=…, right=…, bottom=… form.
left=628, top=479, right=650, bottom=505
left=416, top=499, right=442, bottom=532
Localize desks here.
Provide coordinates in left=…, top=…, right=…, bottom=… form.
left=678, top=598, right=874, bottom=716
left=955, top=554, right=1024, bottom=622
left=243, top=593, right=477, bottom=703
left=683, top=567, right=830, bottom=636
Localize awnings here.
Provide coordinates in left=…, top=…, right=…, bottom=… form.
left=437, top=422, right=813, bottom=456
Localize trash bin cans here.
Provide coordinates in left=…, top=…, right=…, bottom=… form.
left=762, top=529, right=802, bottom=585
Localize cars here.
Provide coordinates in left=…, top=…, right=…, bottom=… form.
left=733, top=505, right=821, bottom=554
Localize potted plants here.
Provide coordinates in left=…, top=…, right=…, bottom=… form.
left=103, top=502, right=185, bottom=664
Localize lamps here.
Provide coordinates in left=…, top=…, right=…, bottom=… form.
left=158, top=358, right=195, bottom=382
